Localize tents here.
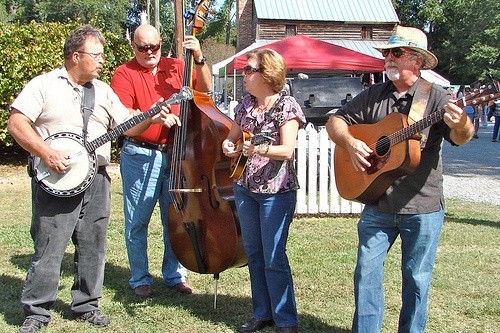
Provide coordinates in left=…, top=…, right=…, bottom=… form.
left=224, top=34, right=386, bottom=108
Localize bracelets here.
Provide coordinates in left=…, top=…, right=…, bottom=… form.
left=262, top=146, right=270, bottom=156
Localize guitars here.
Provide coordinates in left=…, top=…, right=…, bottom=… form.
left=229, top=130, right=276, bottom=182
left=334, top=79, right=500, bottom=202
left=33, top=85, right=195, bottom=198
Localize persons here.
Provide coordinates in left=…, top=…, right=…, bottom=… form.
left=7, top=24, right=172, bottom=333
left=112, top=24, right=212, bottom=298
left=325, top=26, right=476, bottom=333
left=448, top=78, right=500, bottom=142
left=222, top=48, right=306, bottom=333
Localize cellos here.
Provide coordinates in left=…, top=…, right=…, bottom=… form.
left=168, top=0, right=248, bottom=310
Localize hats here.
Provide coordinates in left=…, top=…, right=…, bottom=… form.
left=371, top=24, right=439, bottom=71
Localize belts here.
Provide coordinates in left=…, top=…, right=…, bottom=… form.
left=124, top=136, right=167, bottom=153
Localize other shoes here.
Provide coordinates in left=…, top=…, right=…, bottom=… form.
left=74, top=309, right=112, bottom=327
left=134, top=284, right=153, bottom=297
left=175, top=283, right=193, bottom=295
left=473, top=135, right=479, bottom=138
left=235, top=318, right=274, bottom=333
left=19, top=318, right=48, bottom=333
left=491, top=138, right=496, bottom=142
left=276, top=325, right=297, bottom=333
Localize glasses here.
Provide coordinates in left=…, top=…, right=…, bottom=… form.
left=77, top=51, right=106, bottom=61
left=134, top=41, right=161, bottom=53
left=381, top=47, right=418, bottom=58
left=465, top=91, right=469, bottom=93
left=243, top=65, right=264, bottom=76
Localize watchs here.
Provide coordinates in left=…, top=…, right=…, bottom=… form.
left=194, top=55, right=206, bottom=65
